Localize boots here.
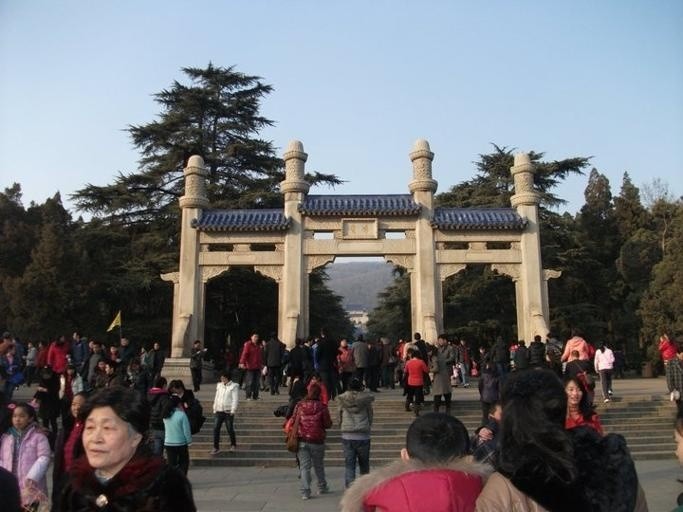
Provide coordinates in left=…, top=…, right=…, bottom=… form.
left=404, top=398, right=413, bottom=411
left=414, top=403, right=421, bottom=417
left=434, top=403, right=440, bottom=412
left=445, top=403, right=451, bottom=416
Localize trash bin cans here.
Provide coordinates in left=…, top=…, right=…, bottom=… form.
left=640, top=361, right=654, bottom=378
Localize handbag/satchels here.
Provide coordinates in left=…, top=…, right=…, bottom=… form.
left=470, top=367, right=478, bottom=376
left=286, top=416, right=299, bottom=453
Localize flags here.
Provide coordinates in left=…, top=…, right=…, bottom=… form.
left=106, top=310, right=122, bottom=331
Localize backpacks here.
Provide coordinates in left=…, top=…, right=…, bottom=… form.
left=183, top=398, right=207, bottom=434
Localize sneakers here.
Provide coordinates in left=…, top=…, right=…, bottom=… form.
left=607, top=389, right=613, bottom=396
left=301, top=493, right=312, bottom=500
left=230, top=445, right=235, bottom=452
left=318, top=487, right=329, bottom=494
left=603, top=398, right=610, bottom=404
left=210, top=448, right=221, bottom=456
